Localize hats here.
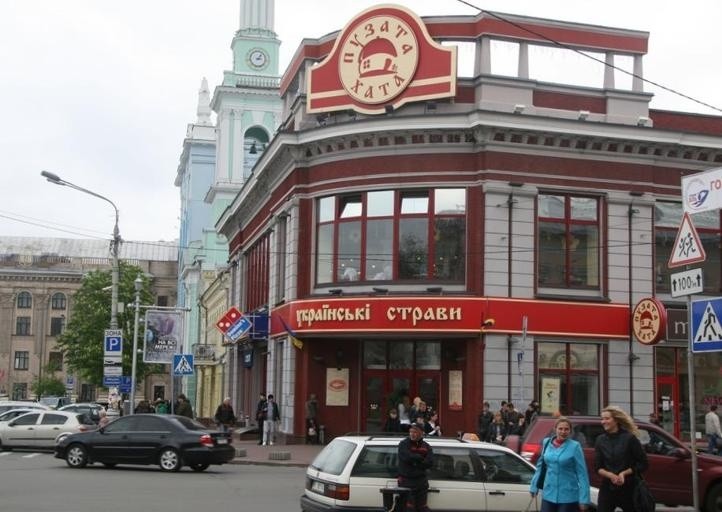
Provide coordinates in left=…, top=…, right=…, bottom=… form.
left=408, top=422, right=424, bottom=431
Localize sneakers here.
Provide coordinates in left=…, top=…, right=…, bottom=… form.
left=262, top=441, right=274, bottom=447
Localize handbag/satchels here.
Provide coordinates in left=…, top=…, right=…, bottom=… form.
left=537, top=439, right=551, bottom=490
left=625, top=476, right=655, bottom=511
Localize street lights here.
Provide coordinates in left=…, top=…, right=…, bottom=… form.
left=129, top=271, right=140, bottom=408
left=37, top=163, right=121, bottom=420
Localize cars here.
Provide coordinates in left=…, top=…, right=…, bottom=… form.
left=54, top=412, right=235, bottom=470
left=0, top=394, right=107, bottom=428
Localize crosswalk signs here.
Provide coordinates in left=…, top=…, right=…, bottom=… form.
left=688, top=298, right=722, bottom=352
left=175, top=353, right=194, bottom=375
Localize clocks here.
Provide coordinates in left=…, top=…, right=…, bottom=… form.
left=245, top=47, right=270, bottom=71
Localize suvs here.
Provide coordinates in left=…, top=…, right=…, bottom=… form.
left=300, top=432, right=602, bottom=512
left=507, top=412, right=721, bottom=511
left=1, top=411, right=96, bottom=450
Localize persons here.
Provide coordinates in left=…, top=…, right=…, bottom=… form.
left=214, top=396, right=235, bottom=432
left=530, top=419, right=591, bottom=512
left=261, top=394, right=281, bottom=446
left=384, top=395, right=541, bottom=445
left=256, top=393, right=267, bottom=445
left=705, top=406, right=722, bottom=456
left=304, top=393, right=319, bottom=445
left=594, top=406, right=649, bottom=512
left=134, top=393, right=193, bottom=420
left=398, top=423, right=434, bottom=512
